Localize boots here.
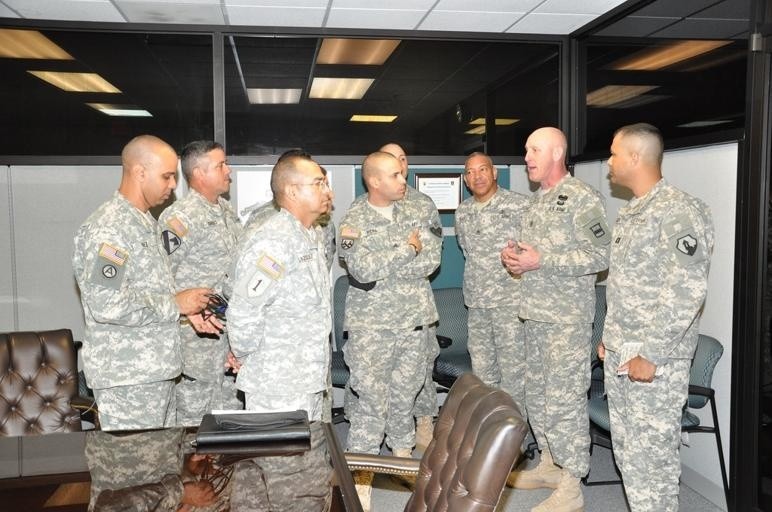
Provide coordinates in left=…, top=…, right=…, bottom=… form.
left=415, top=416, right=435, bottom=453
left=507, top=451, right=563, bottom=489
left=530, top=468, right=584, bottom=512
left=353, top=470, right=375, bottom=512
left=389, top=447, right=417, bottom=491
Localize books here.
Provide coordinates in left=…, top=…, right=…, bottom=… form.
left=196, top=411, right=311, bottom=445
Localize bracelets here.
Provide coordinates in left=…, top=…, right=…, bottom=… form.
left=409, top=243, right=419, bottom=256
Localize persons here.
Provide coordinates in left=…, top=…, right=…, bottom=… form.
left=337, top=151, right=442, bottom=512
left=246, top=166, right=336, bottom=422
left=455, top=153, right=529, bottom=439
left=598, top=123, right=714, bottom=512
left=159, top=141, right=245, bottom=426
left=380, top=143, right=443, bottom=451
left=72, top=135, right=223, bottom=432
left=225, top=151, right=334, bottom=512
left=84, top=421, right=334, bottom=512
left=501, top=127, right=611, bottom=512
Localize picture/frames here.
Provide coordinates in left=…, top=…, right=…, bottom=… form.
left=413, top=172, right=464, bottom=214
